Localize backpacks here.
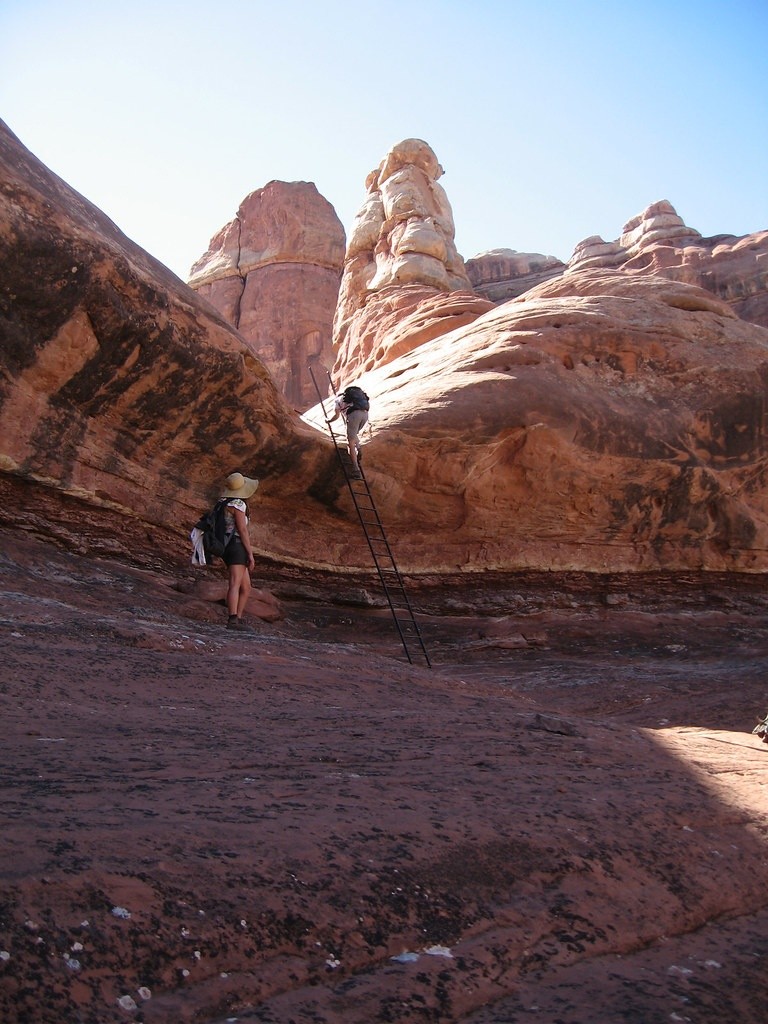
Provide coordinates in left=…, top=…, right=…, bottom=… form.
left=197, top=497, right=237, bottom=558
left=336, top=385, right=370, bottom=414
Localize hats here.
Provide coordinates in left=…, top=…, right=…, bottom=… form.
left=216, top=472, right=259, bottom=499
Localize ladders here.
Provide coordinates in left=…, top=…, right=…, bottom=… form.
left=307, top=365, right=432, bottom=668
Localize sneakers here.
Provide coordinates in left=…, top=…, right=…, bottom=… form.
left=225, top=615, right=255, bottom=635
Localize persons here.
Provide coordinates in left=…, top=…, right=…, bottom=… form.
left=325, top=386, right=369, bottom=478
left=214, top=472, right=255, bottom=629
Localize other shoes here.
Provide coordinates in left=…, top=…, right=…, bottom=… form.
left=356, top=448, right=362, bottom=461
left=352, top=467, right=361, bottom=480
